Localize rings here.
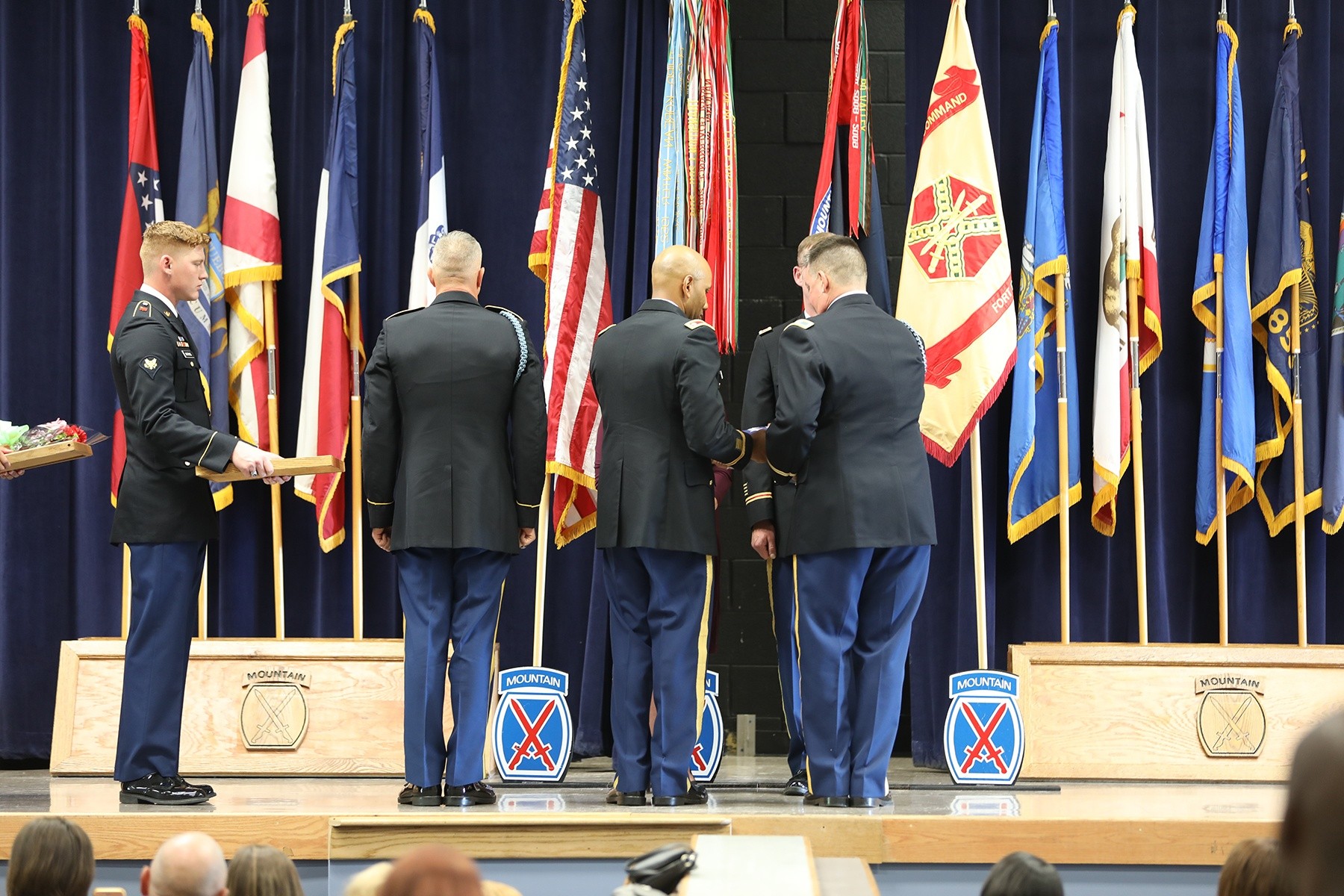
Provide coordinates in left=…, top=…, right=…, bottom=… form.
left=250, top=471, right=257, bottom=477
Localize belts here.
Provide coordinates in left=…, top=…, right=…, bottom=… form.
left=119, top=772, right=208, bottom=804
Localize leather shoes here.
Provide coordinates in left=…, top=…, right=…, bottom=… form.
left=398, top=783, right=442, bottom=806
left=850, top=791, right=892, bottom=808
left=605, top=785, right=646, bottom=805
left=653, top=785, right=708, bottom=806
left=172, top=773, right=216, bottom=798
left=803, top=792, right=848, bottom=807
left=443, top=781, right=496, bottom=806
left=783, top=771, right=808, bottom=795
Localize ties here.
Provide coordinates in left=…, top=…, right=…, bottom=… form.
left=178, top=314, right=184, bottom=330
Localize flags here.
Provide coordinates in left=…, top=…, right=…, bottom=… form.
left=529, top=0, right=613, bottom=551
left=801, top=0, right=892, bottom=317
left=1192, top=19, right=1255, bottom=547
left=294, top=20, right=367, bottom=553
left=1251, top=24, right=1321, bottom=538
left=107, top=15, right=166, bottom=510
left=1090, top=5, right=1163, bottom=538
left=895, top=0, right=1017, bottom=468
left=222, top=0, right=283, bottom=451
left=175, top=12, right=235, bottom=512
left=1006, top=21, right=1082, bottom=545
left=407, top=9, right=449, bottom=312
left=656, top=1, right=739, bottom=356
left=1321, top=213, right=1344, bottom=534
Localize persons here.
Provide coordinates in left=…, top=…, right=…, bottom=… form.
left=590, top=246, right=766, bottom=805
left=741, top=232, right=837, bottom=796
left=7, top=709, right=1344, bottom=896
left=361, top=231, right=547, bottom=808
left=111, top=218, right=291, bottom=807
left=765, top=236, right=939, bottom=808
